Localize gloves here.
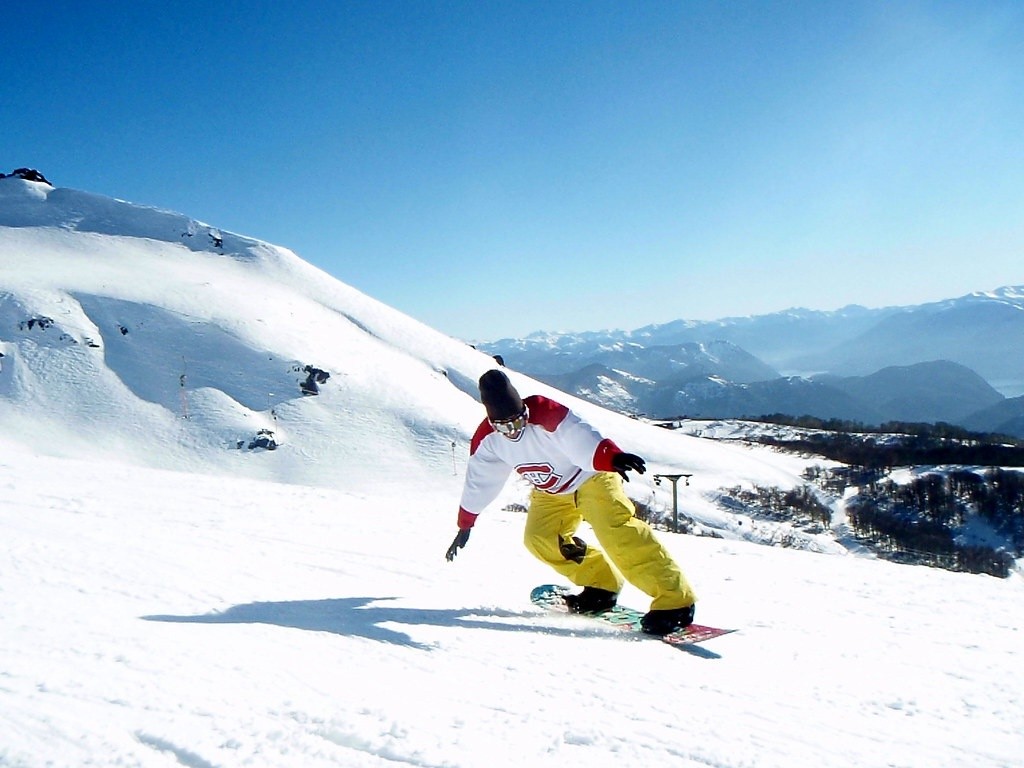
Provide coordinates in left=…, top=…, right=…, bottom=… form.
left=612, top=452, right=646, bottom=482
left=446, top=529, right=471, bottom=562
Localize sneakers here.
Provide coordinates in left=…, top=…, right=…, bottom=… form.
left=565, top=586, right=618, bottom=613
left=640, top=603, right=695, bottom=634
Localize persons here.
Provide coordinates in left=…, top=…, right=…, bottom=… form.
left=445, top=369, right=699, bottom=636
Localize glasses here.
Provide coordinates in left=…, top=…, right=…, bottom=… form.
left=488, top=404, right=529, bottom=433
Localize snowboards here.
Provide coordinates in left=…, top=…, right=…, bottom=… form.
left=530, top=582, right=741, bottom=647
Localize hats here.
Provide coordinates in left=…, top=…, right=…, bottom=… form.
left=478, top=369, right=523, bottom=421
